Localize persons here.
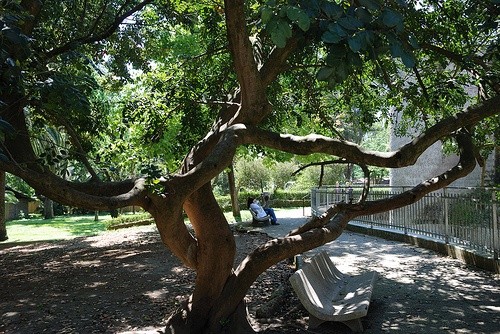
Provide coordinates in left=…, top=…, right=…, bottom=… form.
left=247, top=197, right=270, bottom=220
left=263, top=196, right=279, bottom=225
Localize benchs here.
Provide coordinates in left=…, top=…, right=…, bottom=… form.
left=290, top=248, right=376, bottom=332
left=249, top=208, right=271, bottom=227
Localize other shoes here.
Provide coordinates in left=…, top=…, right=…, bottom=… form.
left=271, top=222, right=280, bottom=225
